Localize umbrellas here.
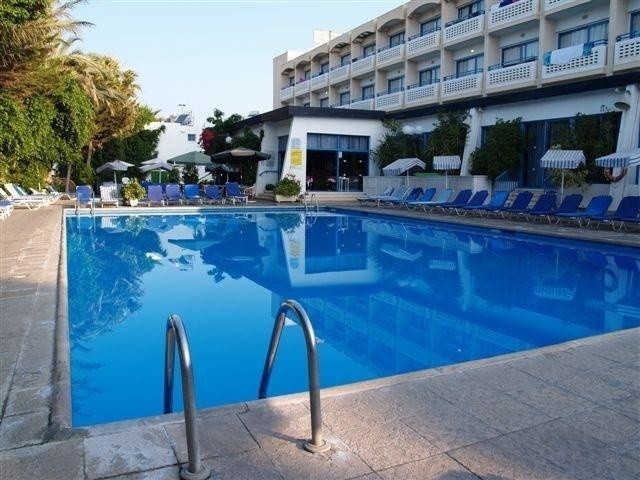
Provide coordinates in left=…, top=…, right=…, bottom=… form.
left=382, top=157, right=426, bottom=191
left=205, top=161, right=244, bottom=184
left=539, top=149, right=586, bottom=202
left=217, top=243, right=271, bottom=261
left=167, top=234, right=226, bottom=251
left=430, top=241, right=455, bottom=271
left=168, top=150, right=221, bottom=185
left=97, top=157, right=179, bottom=203
left=212, top=144, right=271, bottom=203
left=595, top=149, right=640, bottom=170
left=532, top=249, right=575, bottom=300
left=380, top=233, right=422, bottom=262
left=433, top=155, right=462, bottom=190
left=583, top=298, right=619, bottom=313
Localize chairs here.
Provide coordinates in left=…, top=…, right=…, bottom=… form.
left=354, top=185, right=639, bottom=235
left=75, top=179, right=250, bottom=207
left=0, top=182, right=72, bottom=222
